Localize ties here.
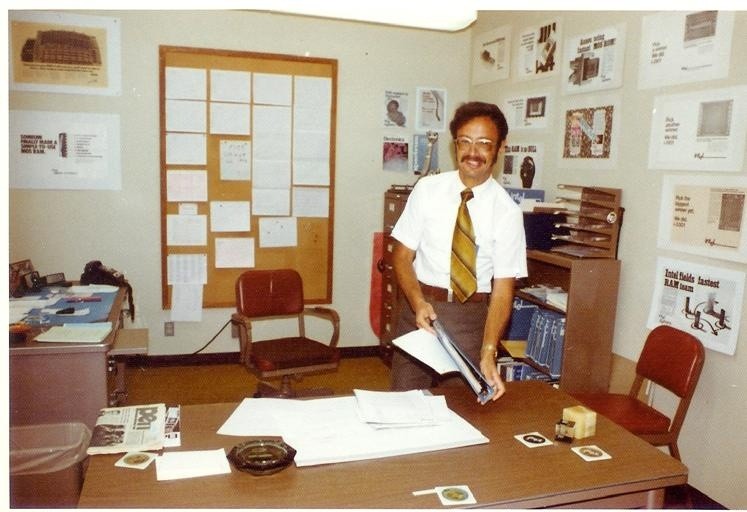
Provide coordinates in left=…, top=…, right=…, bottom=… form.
left=449, top=188, right=478, bottom=303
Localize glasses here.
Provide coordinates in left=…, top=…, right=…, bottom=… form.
left=453, top=136, right=497, bottom=148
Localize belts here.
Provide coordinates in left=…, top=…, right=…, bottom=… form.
left=418, top=280, right=489, bottom=303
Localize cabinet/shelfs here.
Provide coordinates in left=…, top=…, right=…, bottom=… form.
left=494, top=247, right=621, bottom=395
left=550, top=183, right=622, bottom=259
left=9, top=280, right=150, bottom=434
left=379, top=191, right=412, bottom=368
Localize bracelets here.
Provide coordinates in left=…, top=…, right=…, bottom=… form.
left=480, top=343, right=498, bottom=363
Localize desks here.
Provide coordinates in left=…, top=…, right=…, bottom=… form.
left=75, top=380, right=689, bottom=509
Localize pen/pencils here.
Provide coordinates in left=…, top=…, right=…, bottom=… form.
left=66, top=297, right=101, bottom=302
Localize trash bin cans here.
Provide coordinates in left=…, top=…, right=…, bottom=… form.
left=8, top=422, right=92, bottom=510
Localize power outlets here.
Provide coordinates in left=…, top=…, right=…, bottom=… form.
left=165, top=322, right=174, bottom=337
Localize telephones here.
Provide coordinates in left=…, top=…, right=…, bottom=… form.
left=80, top=261, right=125, bottom=286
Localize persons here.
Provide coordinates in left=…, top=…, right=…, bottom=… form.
left=389, top=100, right=529, bottom=406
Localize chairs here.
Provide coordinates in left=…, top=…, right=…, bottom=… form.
left=228, top=267, right=341, bottom=399
left=565, top=325, right=705, bottom=509
